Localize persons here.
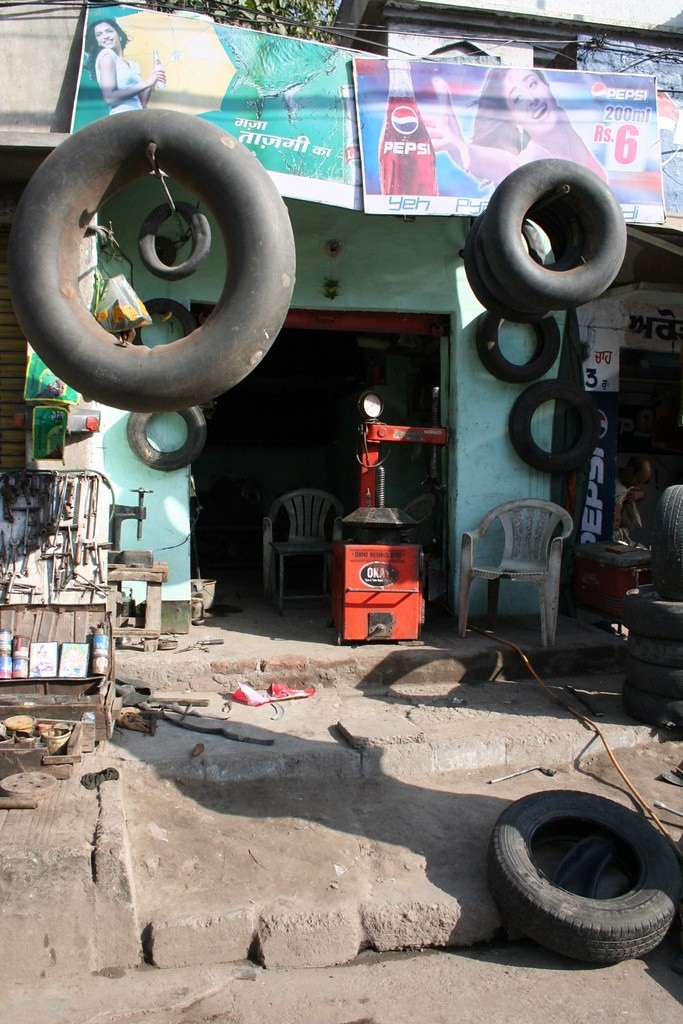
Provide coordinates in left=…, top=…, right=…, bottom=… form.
left=613, top=456, right=651, bottom=544
left=83, top=18, right=167, bottom=116
left=418, top=68, right=608, bottom=189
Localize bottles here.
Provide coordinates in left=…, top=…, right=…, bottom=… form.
left=13, top=635, right=31, bottom=657
left=12, top=656, right=30, bottom=679
left=92, top=634, right=111, bottom=657
left=0, top=629, right=12, bottom=654
left=378, top=60, right=437, bottom=198
left=91, top=655, right=110, bottom=675
left=152, top=50, right=165, bottom=88
left=0, top=654, right=13, bottom=679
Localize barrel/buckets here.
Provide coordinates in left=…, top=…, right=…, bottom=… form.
left=191, top=579, right=217, bottom=609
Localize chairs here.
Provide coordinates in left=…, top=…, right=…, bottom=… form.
left=262, top=488, right=344, bottom=615
left=459, top=497, right=575, bottom=646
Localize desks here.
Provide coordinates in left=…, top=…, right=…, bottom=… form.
left=108, top=562, right=173, bottom=652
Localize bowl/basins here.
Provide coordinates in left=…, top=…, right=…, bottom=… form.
left=41, top=728, right=71, bottom=754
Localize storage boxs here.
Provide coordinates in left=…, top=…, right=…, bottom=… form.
left=573, top=540, right=656, bottom=618
